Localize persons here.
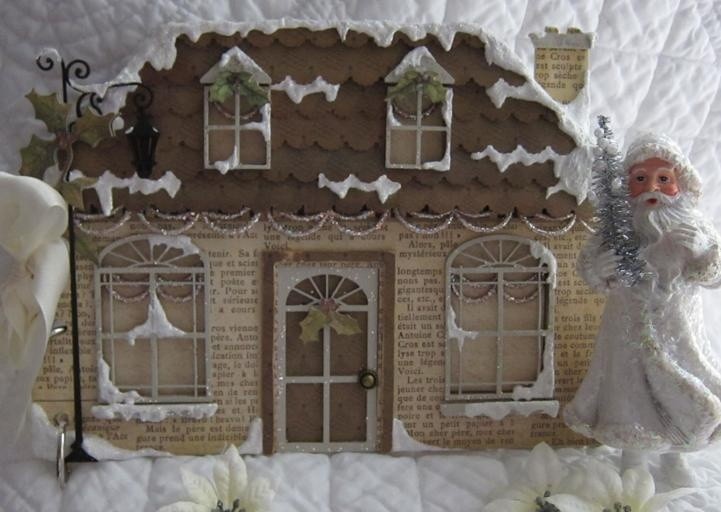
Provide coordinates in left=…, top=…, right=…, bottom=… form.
left=562, top=112, right=721, bottom=453
